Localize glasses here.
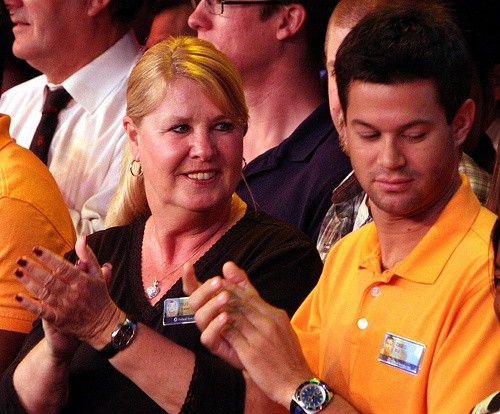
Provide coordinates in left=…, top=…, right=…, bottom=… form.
left=191, top=0, right=291, bottom=15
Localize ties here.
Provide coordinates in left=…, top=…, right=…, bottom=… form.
left=29, top=85, right=73, bottom=166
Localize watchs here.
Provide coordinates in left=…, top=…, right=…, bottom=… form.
left=99, top=314, right=138, bottom=360
left=289, top=376, right=334, bottom=414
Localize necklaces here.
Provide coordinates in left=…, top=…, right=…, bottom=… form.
left=147, top=224, right=202, bottom=300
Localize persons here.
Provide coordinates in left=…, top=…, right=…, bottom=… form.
left=182, top=0, right=500, bottom=414
left=0, top=0, right=500, bottom=263
left=0, top=112, right=76, bottom=371
left=0, top=35, right=323, bottom=414
left=469, top=218, right=500, bottom=414
left=167, top=301, right=177, bottom=317
left=379, top=335, right=394, bottom=357
